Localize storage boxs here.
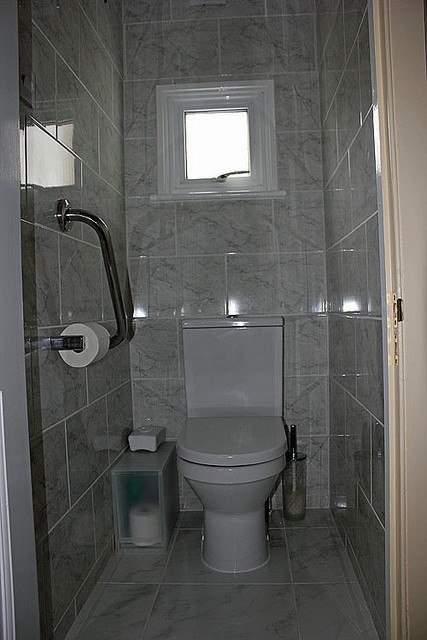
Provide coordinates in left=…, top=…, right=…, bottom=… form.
left=110, top=439, right=180, bottom=554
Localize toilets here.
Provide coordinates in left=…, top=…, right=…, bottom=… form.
left=175, top=315, right=288, bottom=574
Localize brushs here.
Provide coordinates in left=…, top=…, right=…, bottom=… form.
left=286, top=424, right=303, bottom=515
